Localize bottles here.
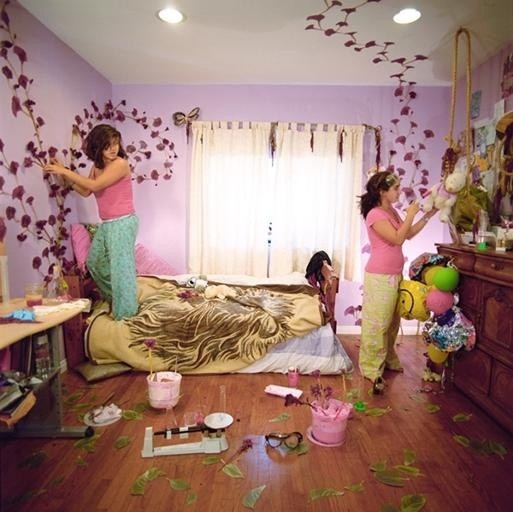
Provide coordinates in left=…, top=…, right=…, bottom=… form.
left=494, top=232, right=506, bottom=253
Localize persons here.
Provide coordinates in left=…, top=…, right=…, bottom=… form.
left=355, top=171, right=440, bottom=395
left=43, top=124, right=140, bottom=321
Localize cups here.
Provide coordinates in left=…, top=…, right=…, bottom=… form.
left=477, top=225, right=487, bottom=250
left=288, top=367, right=298, bottom=390
left=25, top=282, right=44, bottom=307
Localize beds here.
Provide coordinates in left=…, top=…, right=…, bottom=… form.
left=58, top=251, right=354, bottom=375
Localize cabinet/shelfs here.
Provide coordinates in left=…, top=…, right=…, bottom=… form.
left=434, top=235, right=512, bottom=425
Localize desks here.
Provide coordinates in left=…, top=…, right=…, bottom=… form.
left=0, top=291, right=93, bottom=437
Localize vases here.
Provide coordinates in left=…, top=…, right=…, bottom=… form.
left=305, top=399, right=350, bottom=446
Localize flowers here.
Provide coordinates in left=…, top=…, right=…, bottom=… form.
left=283, top=369, right=360, bottom=421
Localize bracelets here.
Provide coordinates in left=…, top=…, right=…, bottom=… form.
left=70, top=182, right=75, bottom=187
left=422, top=216, right=429, bottom=222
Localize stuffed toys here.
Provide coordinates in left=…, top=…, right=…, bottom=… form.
left=418, top=182, right=457, bottom=223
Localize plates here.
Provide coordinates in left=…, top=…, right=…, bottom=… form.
left=203, top=412, right=234, bottom=429
